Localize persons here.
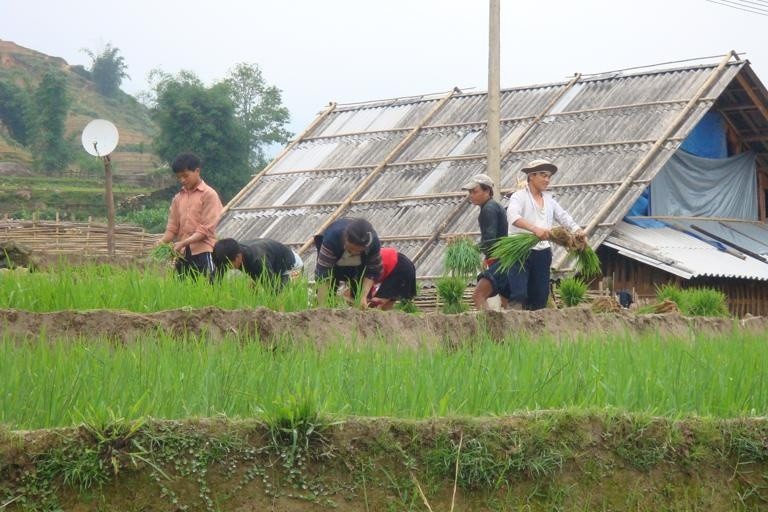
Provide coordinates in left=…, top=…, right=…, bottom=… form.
left=463, top=175, right=508, bottom=268
left=312, top=217, right=384, bottom=308
left=312, top=235, right=417, bottom=311
left=505, top=160, right=587, bottom=309
left=158, top=154, right=225, bottom=286
left=473, top=257, right=511, bottom=312
left=211, top=238, right=306, bottom=297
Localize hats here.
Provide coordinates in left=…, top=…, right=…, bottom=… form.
left=521, top=160, right=557, bottom=175
left=461, top=175, right=494, bottom=189
